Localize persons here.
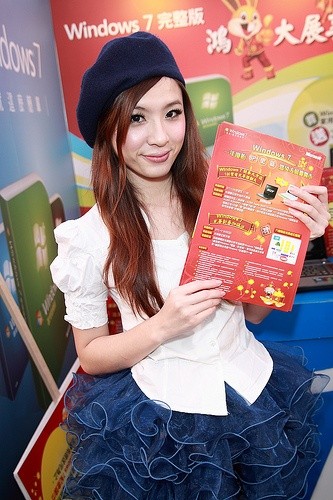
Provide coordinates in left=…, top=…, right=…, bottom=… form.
left=47, top=30, right=331, bottom=500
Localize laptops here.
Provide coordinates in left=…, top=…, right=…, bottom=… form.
left=295, top=236, right=333, bottom=294
left=256, top=184, right=279, bottom=201
left=278, top=185, right=299, bottom=201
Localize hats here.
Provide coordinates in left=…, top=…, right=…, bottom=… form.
left=77, top=30, right=186, bottom=149
